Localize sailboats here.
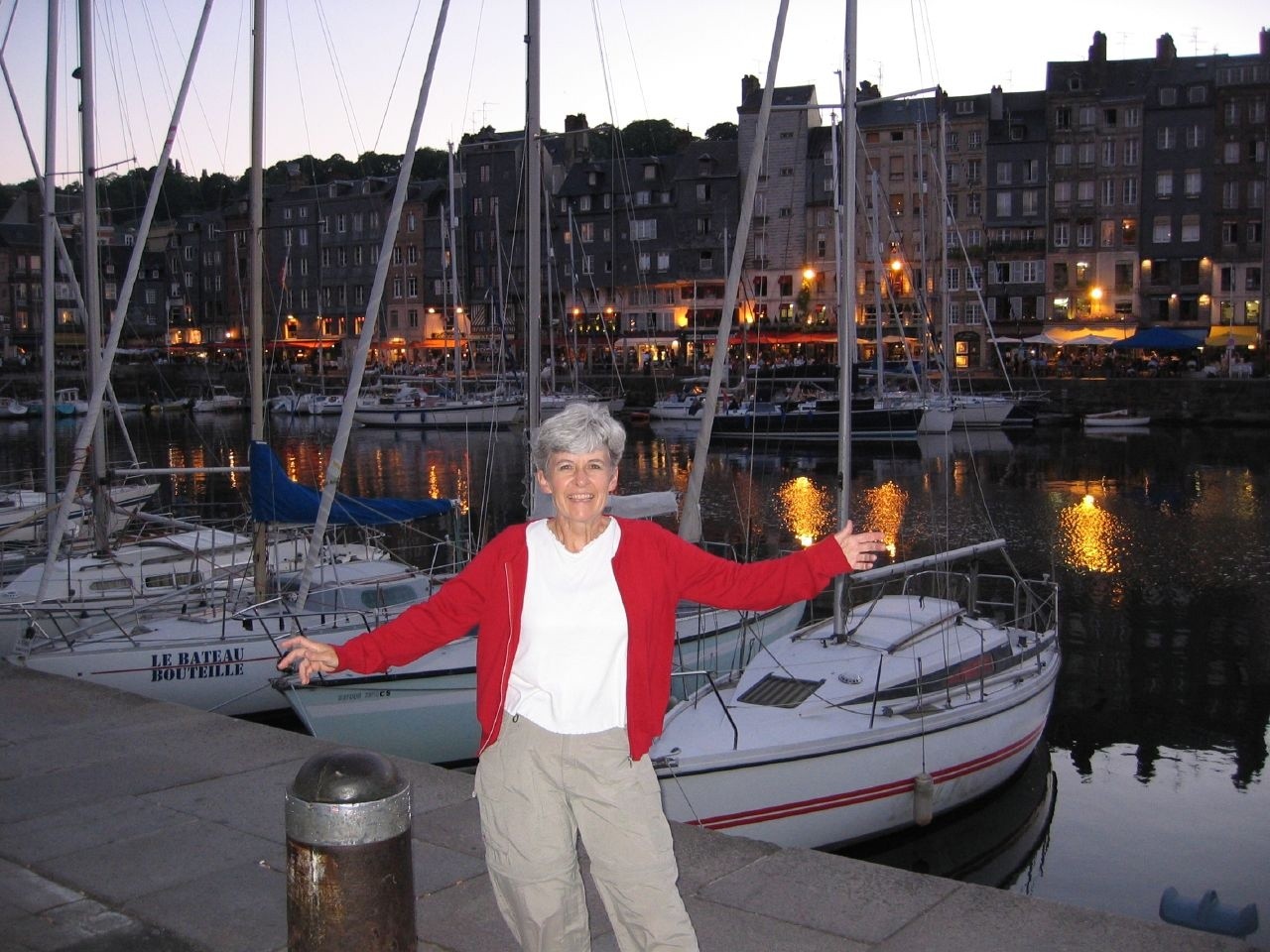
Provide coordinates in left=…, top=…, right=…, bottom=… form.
left=0, top=1, right=1155, bottom=849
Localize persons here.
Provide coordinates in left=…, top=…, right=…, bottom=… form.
left=1013, top=352, right=1252, bottom=377
left=390, top=355, right=472, bottom=375
left=278, top=401, right=885, bottom=952
left=643, top=349, right=828, bottom=376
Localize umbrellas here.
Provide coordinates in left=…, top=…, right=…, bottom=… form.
left=701, top=334, right=918, bottom=359
left=1205, top=332, right=1257, bottom=355
left=1023, top=333, right=1066, bottom=354
left=986, top=336, right=1023, bottom=343
left=1064, top=334, right=1116, bottom=364
left=1113, top=329, right=1206, bottom=359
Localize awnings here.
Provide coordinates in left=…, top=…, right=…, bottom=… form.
left=615, top=338, right=679, bottom=346
left=207, top=341, right=339, bottom=348
left=1180, top=330, right=1208, bottom=341
left=413, top=339, right=468, bottom=347
left=1042, top=326, right=1136, bottom=341
left=1210, top=326, right=1257, bottom=336
